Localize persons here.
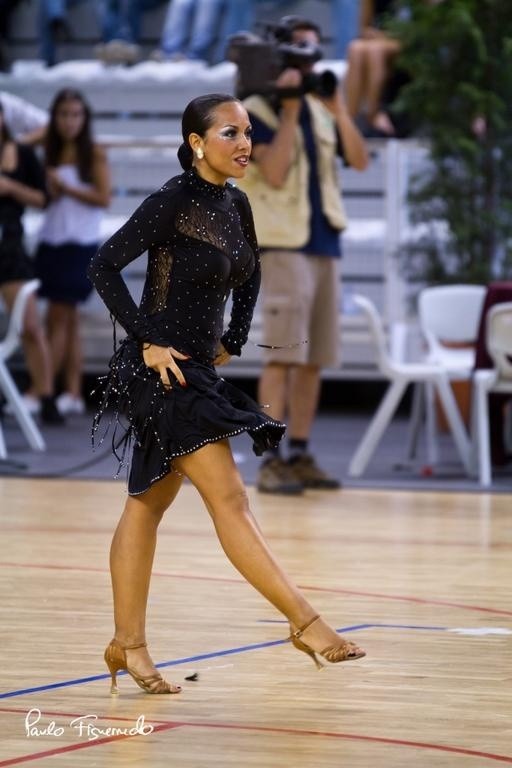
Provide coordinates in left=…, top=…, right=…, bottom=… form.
left=1, top=89, right=109, bottom=418
left=0, top=88, right=52, bottom=149
left=87, top=94, right=367, bottom=695
left=36, top=1, right=489, bottom=139
left=1, top=102, right=65, bottom=423
left=238, top=14, right=370, bottom=493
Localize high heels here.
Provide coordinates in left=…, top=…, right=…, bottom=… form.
left=288, top=615, right=366, bottom=669
left=104, top=638, right=181, bottom=694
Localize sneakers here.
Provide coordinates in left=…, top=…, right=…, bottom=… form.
left=291, top=453, right=339, bottom=487
left=258, top=457, right=301, bottom=494
left=56, top=393, right=85, bottom=416
left=4, top=392, right=42, bottom=415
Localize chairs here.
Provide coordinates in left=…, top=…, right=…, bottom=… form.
left=348, top=283, right=512, bottom=491
left=0, top=278, right=45, bottom=461
